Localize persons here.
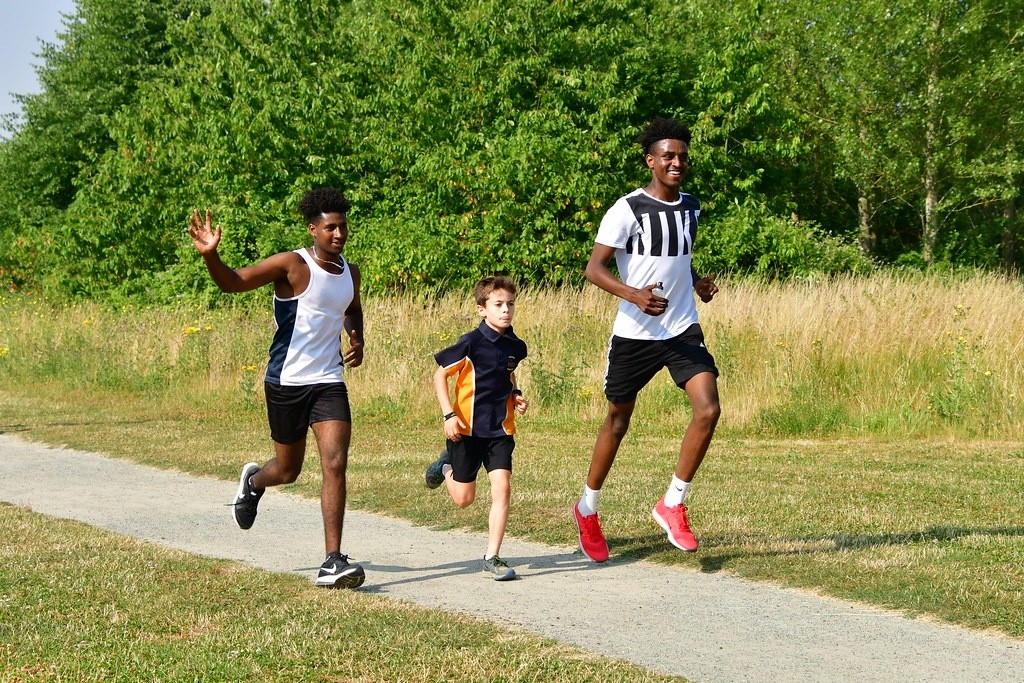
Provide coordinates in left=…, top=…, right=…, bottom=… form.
left=573, top=121, right=719, bottom=563
left=426, top=277, right=529, bottom=583
left=188, top=186, right=365, bottom=590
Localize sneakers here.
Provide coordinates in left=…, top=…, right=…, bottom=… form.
left=651, top=497, right=698, bottom=552
left=224, top=462, right=265, bottom=529
left=574, top=500, right=609, bottom=563
left=482, top=554, right=516, bottom=581
left=314, top=551, right=365, bottom=589
left=426, top=449, right=451, bottom=489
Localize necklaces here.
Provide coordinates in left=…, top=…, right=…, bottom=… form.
left=310, top=246, right=345, bottom=270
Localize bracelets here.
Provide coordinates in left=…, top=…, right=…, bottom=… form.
left=443, top=412, right=456, bottom=421
left=512, top=389, right=522, bottom=396
